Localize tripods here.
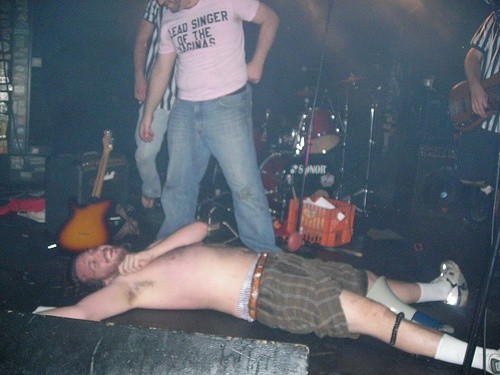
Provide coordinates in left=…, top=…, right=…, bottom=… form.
left=341, top=105, right=375, bottom=216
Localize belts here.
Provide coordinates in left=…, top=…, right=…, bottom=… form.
left=249, top=252, right=268, bottom=319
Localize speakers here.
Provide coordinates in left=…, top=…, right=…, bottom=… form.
left=45, top=151, right=126, bottom=230
left=393, top=88, right=464, bottom=213
left=0, top=308, right=311, bottom=375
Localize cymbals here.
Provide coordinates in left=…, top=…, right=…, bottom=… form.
left=340, top=77, right=369, bottom=83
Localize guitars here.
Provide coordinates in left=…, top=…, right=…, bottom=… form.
left=449, top=71, right=500, bottom=133
left=53, top=126, right=115, bottom=251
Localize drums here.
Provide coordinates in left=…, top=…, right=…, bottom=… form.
left=257, top=152, right=340, bottom=240
left=297, top=106, right=340, bottom=152
left=252, top=124, right=264, bottom=155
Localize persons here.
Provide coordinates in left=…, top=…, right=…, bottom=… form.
left=34, top=221, right=500, bottom=375
left=134, top=0, right=283, bottom=253
left=464, top=10, right=500, bottom=136
left=284, top=68, right=332, bottom=132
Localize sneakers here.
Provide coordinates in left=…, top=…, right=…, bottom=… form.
left=438, top=260, right=468, bottom=308
left=486, top=350, right=500, bottom=375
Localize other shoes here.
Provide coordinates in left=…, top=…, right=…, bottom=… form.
left=140, top=195, right=154, bottom=216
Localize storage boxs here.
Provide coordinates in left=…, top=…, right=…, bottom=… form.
left=287, top=196, right=355, bottom=249
